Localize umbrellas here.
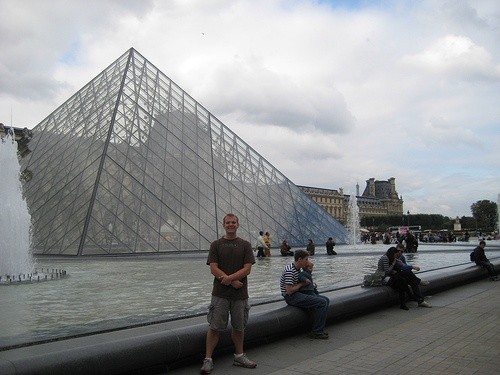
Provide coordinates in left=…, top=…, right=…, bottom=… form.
left=360, top=229, right=369, bottom=232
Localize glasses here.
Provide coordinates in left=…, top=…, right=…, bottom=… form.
left=398, top=250, right=403, bottom=253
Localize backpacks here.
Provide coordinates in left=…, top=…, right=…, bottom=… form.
left=470, top=248, right=477, bottom=261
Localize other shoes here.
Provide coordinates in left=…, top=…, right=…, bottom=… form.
left=421, top=281, right=431, bottom=286
left=312, top=334, right=329, bottom=339
left=400, top=307, right=409, bottom=310
left=322, top=331, right=328, bottom=334
left=419, top=303, right=432, bottom=308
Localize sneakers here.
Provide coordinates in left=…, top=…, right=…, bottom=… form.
left=233, top=353, right=257, bottom=367
left=199, top=359, right=214, bottom=374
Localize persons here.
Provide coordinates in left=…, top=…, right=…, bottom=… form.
left=474, top=240, right=500, bottom=281
left=263, top=232, right=271, bottom=257
left=326, top=237, right=337, bottom=255
left=255, top=231, right=269, bottom=257
left=280, top=250, right=329, bottom=339
left=395, top=244, right=432, bottom=308
left=361, top=231, right=471, bottom=253
left=378, top=247, right=410, bottom=311
left=281, top=239, right=294, bottom=257
left=306, top=239, right=315, bottom=256
left=201, top=214, right=258, bottom=375
left=478, top=231, right=500, bottom=241
left=299, top=261, right=316, bottom=296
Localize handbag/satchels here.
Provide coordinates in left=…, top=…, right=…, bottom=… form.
left=364, top=274, right=382, bottom=286
